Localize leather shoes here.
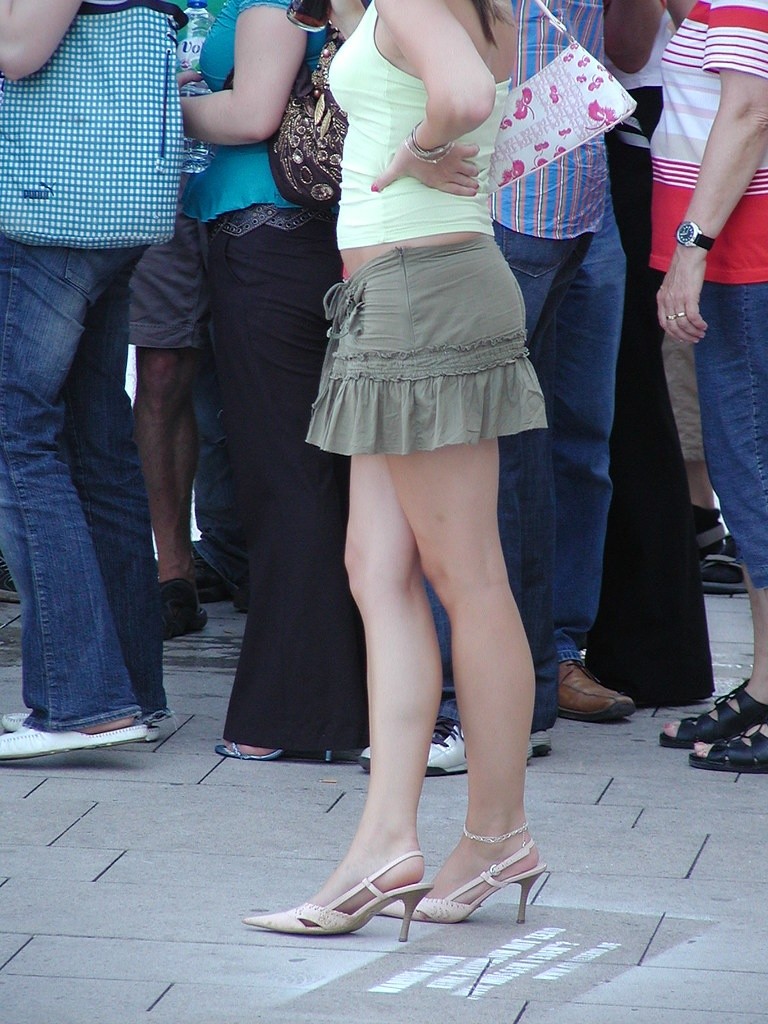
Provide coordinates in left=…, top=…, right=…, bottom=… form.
left=550, top=657, right=634, bottom=723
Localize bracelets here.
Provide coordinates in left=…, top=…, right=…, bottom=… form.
left=404, top=122, right=455, bottom=163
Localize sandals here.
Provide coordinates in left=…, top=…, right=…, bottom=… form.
left=687, top=719, right=768, bottom=773
left=659, top=680, right=768, bottom=750
left=701, top=532, right=748, bottom=590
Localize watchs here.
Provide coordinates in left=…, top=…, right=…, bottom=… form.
left=673, top=221, right=715, bottom=251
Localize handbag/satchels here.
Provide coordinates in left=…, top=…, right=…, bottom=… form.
left=488, top=1, right=637, bottom=194
left=272, top=21, right=350, bottom=206
left=0, top=1, right=184, bottom=250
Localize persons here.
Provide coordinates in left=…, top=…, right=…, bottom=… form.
left=0, top=0, right=185, bottom=759
left=498, top=0, right=665, bottom=721
left=130, top=0, right=534, bottom=774
left=240, top=0, right=549, bottom=942
left=649, top=0, right=768, bottom=773
left=584, top=8, right=715, bottom=705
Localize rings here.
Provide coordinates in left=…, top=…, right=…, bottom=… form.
left=666, top=314, right=677, bottom=320
left=677, top=312, right=686, bottom=317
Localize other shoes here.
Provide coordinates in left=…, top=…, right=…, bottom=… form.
left=158, top=578, right=208, bottom=639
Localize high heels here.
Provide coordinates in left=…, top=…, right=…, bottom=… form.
left=381, top=838, right=546, bottom=925
left=216, top=739, right=333, bottom=761
left=239, top=850, right=434, bottom=942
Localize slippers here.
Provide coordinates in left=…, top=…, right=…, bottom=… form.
left=0, top=726, right=149, bottom=760
left=3, top=714, right=161, bottom=743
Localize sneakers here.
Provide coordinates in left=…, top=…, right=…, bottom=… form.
left=358, top=718, right=533, bottom=775
left=531, top=728, right=554, bottom=756
